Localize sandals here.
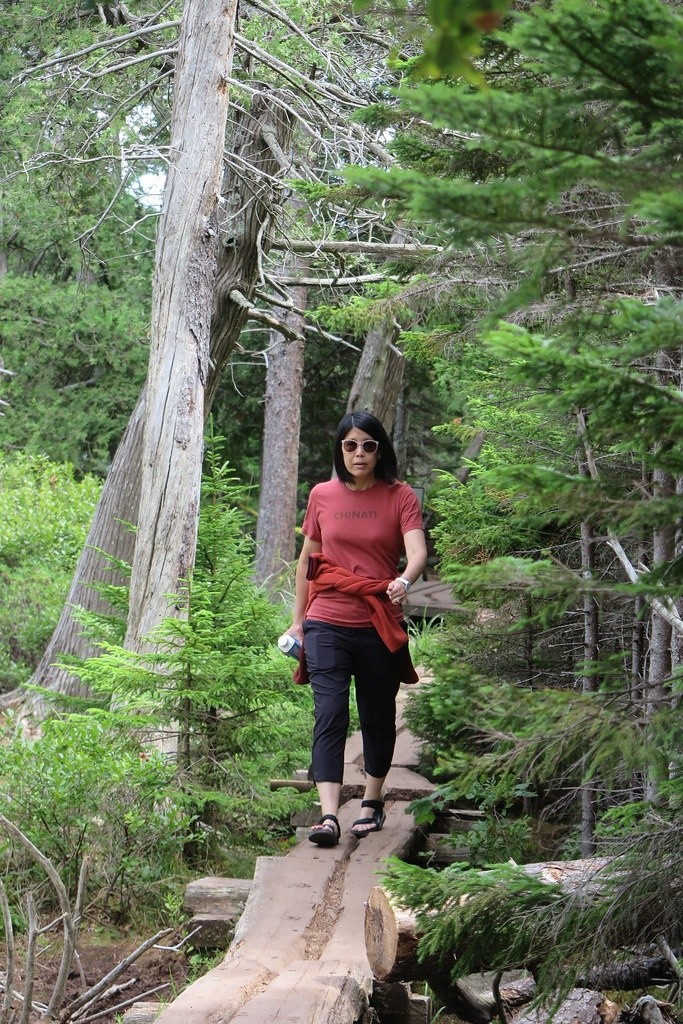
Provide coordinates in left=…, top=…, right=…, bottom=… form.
left=308, top=814, right=341, bottom=847
left=350, top=798, right=387, bottom=835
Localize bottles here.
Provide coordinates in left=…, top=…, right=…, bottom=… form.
left=276, top=635, right=302, bottom=662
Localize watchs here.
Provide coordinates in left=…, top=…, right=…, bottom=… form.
left=397, top=578, right=410, bottom=594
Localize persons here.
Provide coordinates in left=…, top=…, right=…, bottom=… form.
left=278, top=412, right=427, bottom=845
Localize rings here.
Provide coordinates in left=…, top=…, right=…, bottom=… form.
left=396, top=597, right=401, bottom=602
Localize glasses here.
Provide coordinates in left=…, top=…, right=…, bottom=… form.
left=341, top=439, right=379, bottom=455
